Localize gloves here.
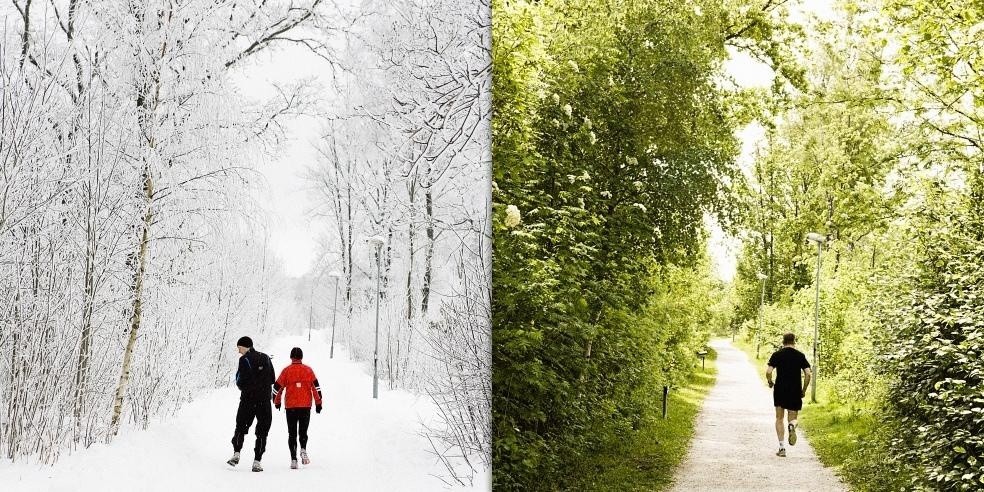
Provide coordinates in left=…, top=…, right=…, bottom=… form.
left=274, top=402, right=281, bottom=411
left=315, top=405, right=322, bottom=414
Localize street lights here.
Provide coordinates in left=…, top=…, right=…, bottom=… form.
left=755, top=273, right=768, bottom=359
left=806, top=232, right=825, bottom=403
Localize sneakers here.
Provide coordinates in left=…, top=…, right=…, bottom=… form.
left=775, top=448, right=786, bottom=457
left=787, top=423, right=796, bottom=446
left=226, top=449, right=310, bottom=471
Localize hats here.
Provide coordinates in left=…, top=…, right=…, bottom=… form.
left=236, top=336, right=252, bottom=348
left=288, top=347, right=303, bottom=359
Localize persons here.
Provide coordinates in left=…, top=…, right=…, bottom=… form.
left=273, top=347, right=322, bottom=469
left=227, top=336, right=275, bottom=472
left=766, top=333, right=811, bottom=456
